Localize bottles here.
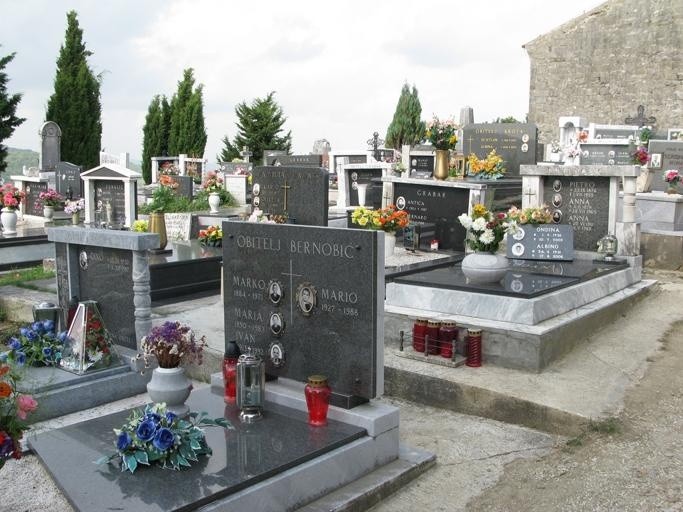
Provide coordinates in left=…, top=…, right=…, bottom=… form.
left=466, top=327, right=482, bottom=367
left=236, top=347, right=265, bottom=421
left=304, top=375, right=331, bottom=428
left=222, top=339, right=241, bottom=404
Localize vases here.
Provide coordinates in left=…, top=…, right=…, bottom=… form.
left=384, top=231, right=397, bottom=258
left=43, top=206, right=54, bottom=223
left=72, top=211, right=80, bottom=225
left=146, top=367, right=193, bottom=417
left=461, top=252, right=510, bottom=285
left=667, top=181, right=679, bottom=194
left=433, top=148, right=450, bottom=181
left=635, top=169, right=655, bottom=192
left=4, top=211, right=19, bottom=235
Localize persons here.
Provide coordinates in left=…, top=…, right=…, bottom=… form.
left=516, top=246, right=521, bottom=253
left=272, top=347, right=280, bottom=364
left=554, top=212, right=560, bottom=222
left=398, top=198, right=405, bottom=208
left=81, top=254, right=85, bottom=266
left=516, top=230, right=522, bottom=238
left=272, top=315, right=280, bottom=332
left=300, top=288, right=312, bottom=311
left=254, top=185, right=258, bottom=194
left=255, top=198, right=258, bottom=205
left=554, top=195, right=560, bottom=206
left=271, top=284, right=280, bottom=301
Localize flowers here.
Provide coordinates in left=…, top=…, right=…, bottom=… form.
left=459, top=204, right=553, bottom=253
left=131, top=322, right=208, bottom=376
left=129, top=160, right=287, bottom=250
left=352, top=205, right=410, bottom=233
left=2, top=296, right=114, bottom=460
left=549, top=131, right=589, bottom=162
left=379, top=153, right=406, bottom=178
left=3, top=183, right=27, bottom=212
left=34, top=188, right=86, bottom=214
left=662, top=170, right=683, bottom=194
left=427, top=119, right=458, bottom=150
left=93, top=401, right=236, bottom=475
left=450, top=148, right=507, bottom=182
left=629, top=128, right=654, bottom=165
